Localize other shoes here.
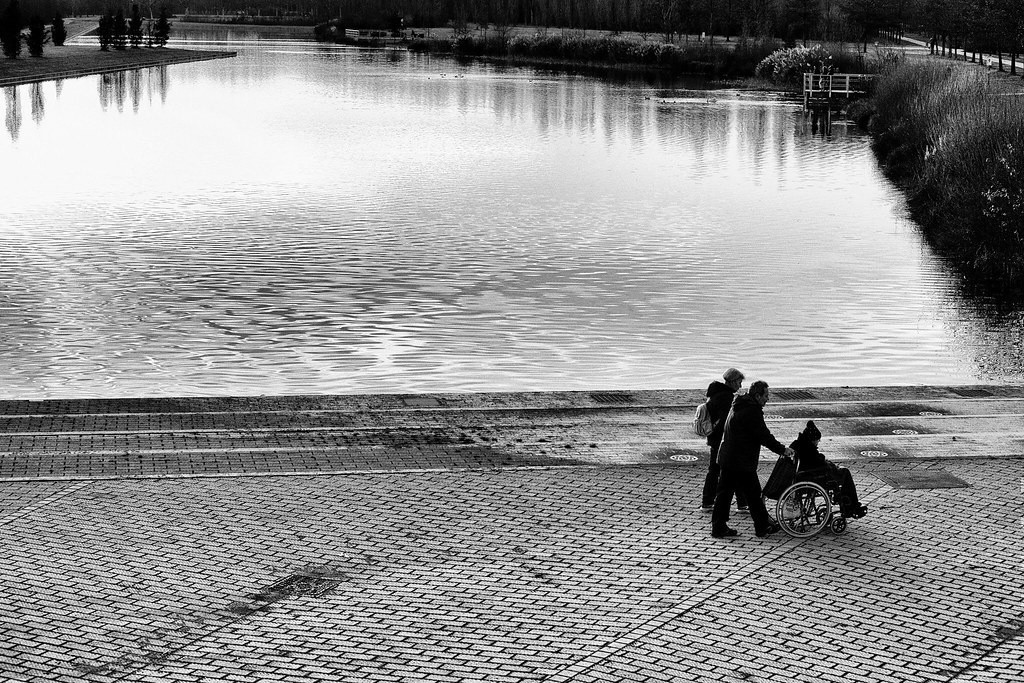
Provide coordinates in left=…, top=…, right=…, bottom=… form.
left=840, top=502, right=868, bottom=518
left=711, top=525, right=738, bottom=537
left=754, top=523, right=780, bottom=537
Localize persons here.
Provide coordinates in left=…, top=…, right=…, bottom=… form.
left=926, top=36, right=933, bottom=49
left=711, top=381, right=792, bottom=538
left=700, top=368, right=750, bottom=512
left=790, top=420, right=868, bottom=518
left=901, top=31, right=904, bottom=37
left=813, top=65, right=834, bottom=90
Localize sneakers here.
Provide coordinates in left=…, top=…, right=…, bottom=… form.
left=700, top=504, right=714, bottom=512
left=736, top=505, right=750, bottom=512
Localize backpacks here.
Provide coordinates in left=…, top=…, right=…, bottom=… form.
left=692, top=397, right=720, bottom=437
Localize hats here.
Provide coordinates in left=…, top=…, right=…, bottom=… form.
left=802, top=420, right=822, bottom=441
left=723, top=367, right=744, bottom=382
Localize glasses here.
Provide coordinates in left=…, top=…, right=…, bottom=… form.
left=818, top=439, right=820, bottom=442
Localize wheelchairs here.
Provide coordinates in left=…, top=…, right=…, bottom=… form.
left=775, top=448, right=857, bottom=538
